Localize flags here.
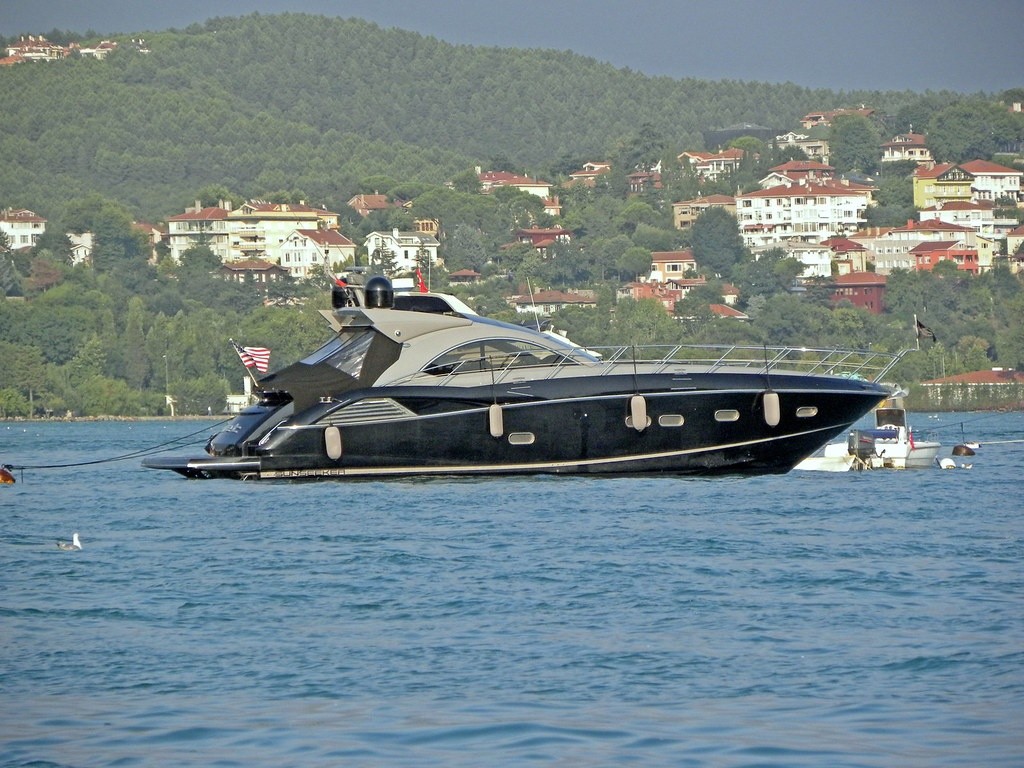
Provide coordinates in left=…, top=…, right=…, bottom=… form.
left=232, top=341, right=273, bottom=375
left=909, top=432, right=916, bottom=453
left=915, top=318, right=937, bottom=343
left=416, top=266, right=428, bottom=294
left=335, top=279, right=347, bottom=288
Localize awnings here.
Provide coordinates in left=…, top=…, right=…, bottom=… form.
left=753, top=225, right=762, bottom=229
left=744, top=224, right=755, bottom=230
left=762, top=224, right=774, bottom=229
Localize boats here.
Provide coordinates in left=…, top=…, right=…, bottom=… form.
left=134, top=273, right=914, bottom=483
left=824, top=401, right=940, bottom=467
left=794, top=453, right=859, bottom=472
